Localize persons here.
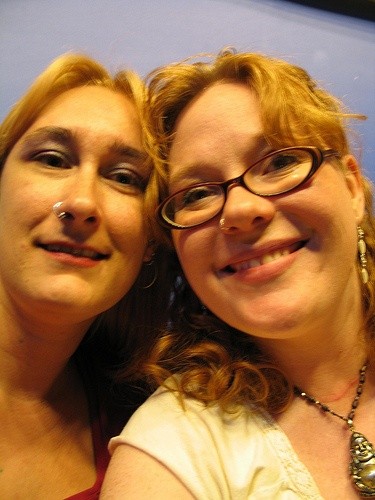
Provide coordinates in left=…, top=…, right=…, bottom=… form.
left=96, top=47, right=375, bottom=500
left=2, top=52, right=180, bottom=500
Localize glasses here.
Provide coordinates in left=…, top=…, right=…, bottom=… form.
left=156, top=144, right=341, bottom=231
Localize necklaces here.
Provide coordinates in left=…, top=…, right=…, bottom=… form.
left=253, top=349, right=374, bottom=500
